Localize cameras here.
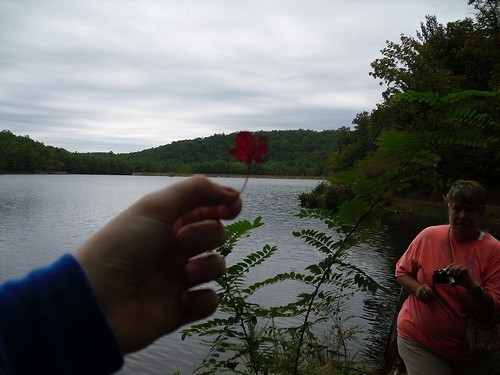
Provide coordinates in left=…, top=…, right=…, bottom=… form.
left=433, top=268, right=457, bottom=287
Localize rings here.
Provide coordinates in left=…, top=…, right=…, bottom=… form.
left=422, top=291, right=426, bottom=298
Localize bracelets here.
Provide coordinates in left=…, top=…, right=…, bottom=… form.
left=467, top=281, right=483, bottom=297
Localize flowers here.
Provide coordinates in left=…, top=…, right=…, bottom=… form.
left=228, top=131, right=268, bottom=194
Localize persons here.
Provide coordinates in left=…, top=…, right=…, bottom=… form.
left=395, top=180, right=500, bottom=375
left=0, top=175, right=242, bottom=375
